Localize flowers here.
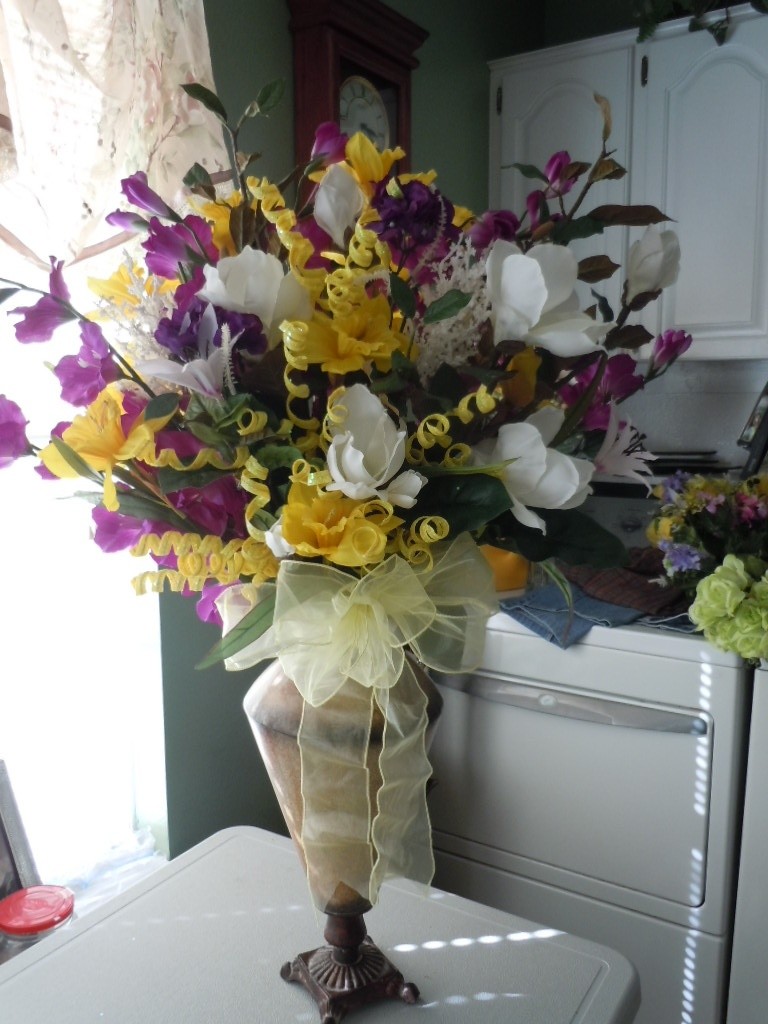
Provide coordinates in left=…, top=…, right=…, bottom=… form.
left=0, top=78, right=688, bottom=604
left=646, top=470, right=767, bottom=666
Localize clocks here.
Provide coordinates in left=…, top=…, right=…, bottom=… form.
left=284, top=0, right=429, bottom=221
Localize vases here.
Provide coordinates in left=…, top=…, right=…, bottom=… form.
left=240, top=592, right=444, bottom=1024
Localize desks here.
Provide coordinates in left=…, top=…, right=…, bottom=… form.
left=0, top=826, right=641, bottom=1023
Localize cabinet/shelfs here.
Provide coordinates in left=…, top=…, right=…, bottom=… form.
left=483, top=0, right=768, bottom=361
